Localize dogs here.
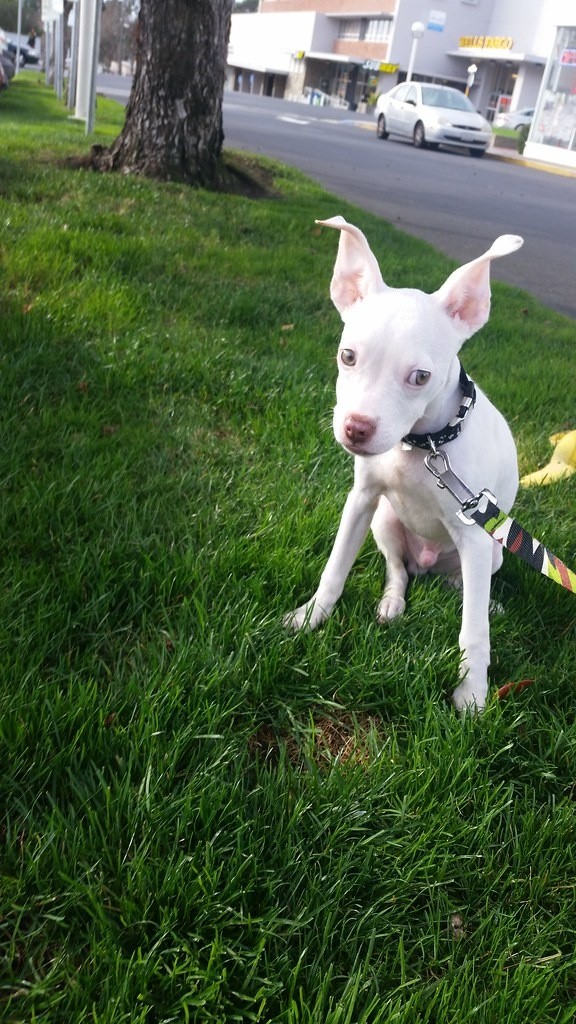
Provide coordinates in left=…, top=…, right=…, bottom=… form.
left=282, top=213, right=525, bottom=719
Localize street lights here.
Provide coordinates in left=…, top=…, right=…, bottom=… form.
left=405, top=21, right=426, bottom=83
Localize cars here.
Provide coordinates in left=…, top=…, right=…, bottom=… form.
left=0, top=41, right=40, bottom=87
left=375, top=80, right=492, bottom=157
